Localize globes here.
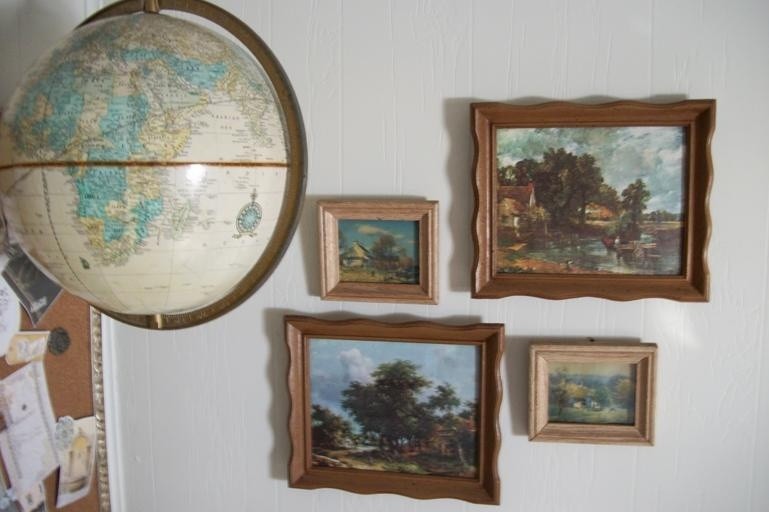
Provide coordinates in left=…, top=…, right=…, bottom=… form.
left=0, top=0, right=309, bottom=332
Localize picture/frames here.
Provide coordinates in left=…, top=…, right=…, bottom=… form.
left=282, top=313, right=505, bottom=506
left=315, top=199, right=441, bottom=306
left=469, top=98, right=717, bottom=303
left=526, top=340, right=658, bottom=447
